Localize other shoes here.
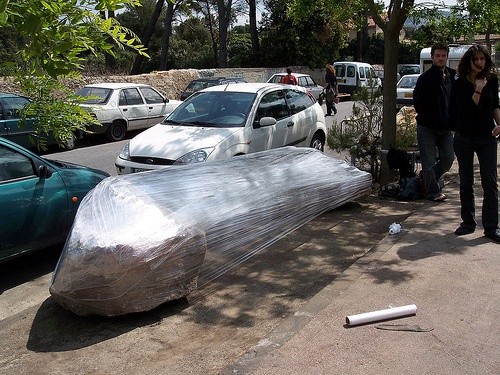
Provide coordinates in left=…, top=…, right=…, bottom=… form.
left=454, top=224, right=475, bottom=236
left=483, top=228, right=500, bottom=242
left=418, top=170, right=427, bottom=196
left=432, top=192, right=446, bottom=201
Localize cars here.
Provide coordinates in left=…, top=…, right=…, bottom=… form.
left=58, top=82, right=196, bottom=142
left=178, top=76, right=248, bottom=103
left=0, top=92, right=47, bottom=148
left=398, top=65, right=421, bottom=77
left=373, top=68, right=402, bottom=84
left=396, top=73, right=422, bottom=103
left=0, top=137, right=112, bottom=266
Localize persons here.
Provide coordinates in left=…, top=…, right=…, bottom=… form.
left=282, top=66, right=297, bottom=85
left=322, top=64, right=338, bottom=116
left=412, top=44, right=455, bottom=201
left=449, top=46, right=500, bottom=244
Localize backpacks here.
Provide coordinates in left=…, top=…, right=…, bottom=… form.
left=286, top=75, right=294, bottom=85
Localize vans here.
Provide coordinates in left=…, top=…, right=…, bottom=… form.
left=419, top=43, right=473, bottom=73
left=332, top=62, right=383, bottom=98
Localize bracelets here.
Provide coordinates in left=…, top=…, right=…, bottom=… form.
left=474, top=91, right=482, bottom=96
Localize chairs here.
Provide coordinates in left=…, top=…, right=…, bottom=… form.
left=348, top=69, right=353, bottom=76
left=207, top=96, right=234, bottom=120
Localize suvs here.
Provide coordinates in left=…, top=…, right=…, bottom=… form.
left=267, top=73, right=326, bottom=104
left=114, top=82, right=328, bottom=178
